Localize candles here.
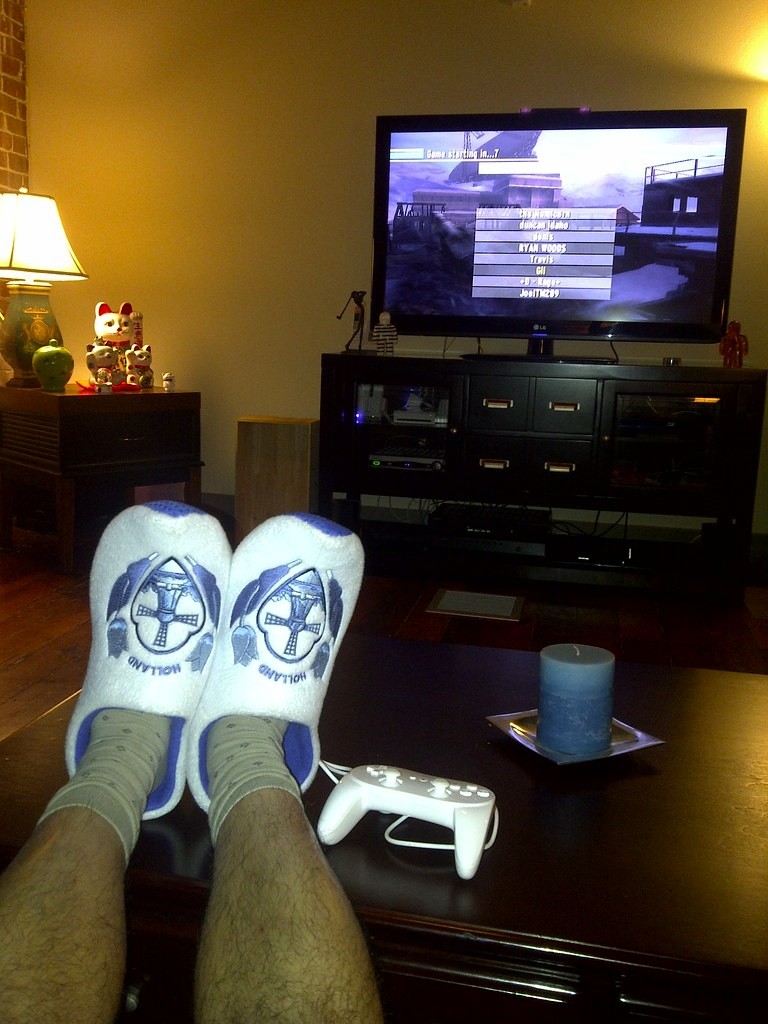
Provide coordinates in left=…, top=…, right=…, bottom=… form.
left=533, top=642, right=615, bottom=756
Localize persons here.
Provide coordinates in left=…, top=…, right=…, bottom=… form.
left=1, top=498, right=391, bottom=1022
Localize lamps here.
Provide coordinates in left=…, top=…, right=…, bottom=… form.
left=0, top=186, right=90, bottom=387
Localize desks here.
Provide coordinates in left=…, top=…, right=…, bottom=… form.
left=0, top=631, right=768, bottom=1022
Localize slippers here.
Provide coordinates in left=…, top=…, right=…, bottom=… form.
left=63, top=499, right=232, bottom=822
left=185, top=512, right=365, bottom=820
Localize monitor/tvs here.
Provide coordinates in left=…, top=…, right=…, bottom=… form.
left=370, top=109, right=746, bottom=343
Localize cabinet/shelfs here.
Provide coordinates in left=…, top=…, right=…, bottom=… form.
left=0, top=383, right=202, bottom=573
left=318, top=350, right=767, bottom=608
left=232, top=415, right=320, bottom=548
